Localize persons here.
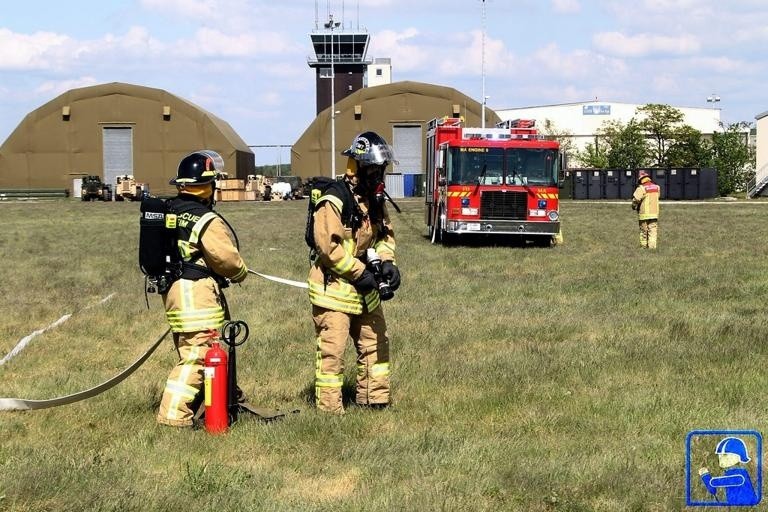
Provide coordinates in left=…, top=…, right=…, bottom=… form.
left=306, top=129, right=403, bottom=416
left=156, top=150, right=249, bottom=429
left=631, top=169, right=662, bottom=250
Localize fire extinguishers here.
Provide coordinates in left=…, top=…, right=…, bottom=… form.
left=197, top=328, right=227, bottom=434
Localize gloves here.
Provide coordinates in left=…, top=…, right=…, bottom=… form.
left=379, top=260, right=400, bottom=291
left=348, top=270, right=378, bottom=290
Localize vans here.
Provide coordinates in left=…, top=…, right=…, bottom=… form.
left=276, top=175, right=306, bottom=200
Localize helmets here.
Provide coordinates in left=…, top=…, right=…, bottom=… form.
left=636, top=172, right=649, bottom=183
left=168, top=149, right=225, bottom=186
left=341, top=132, right=399, bottom=169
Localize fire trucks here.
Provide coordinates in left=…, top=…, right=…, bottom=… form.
left=423, top=114, right=563, bottom=250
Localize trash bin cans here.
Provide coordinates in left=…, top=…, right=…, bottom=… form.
left=404, top=174, right=426, bottom=197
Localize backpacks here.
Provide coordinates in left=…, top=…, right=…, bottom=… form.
left=304, top=175, right=353, bottom=251
left=138, top=191, right=210, bottom=281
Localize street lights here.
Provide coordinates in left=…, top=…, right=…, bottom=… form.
left=706, top=94, right=721, bottom=109
left=324, top=14, right=341, bottom=180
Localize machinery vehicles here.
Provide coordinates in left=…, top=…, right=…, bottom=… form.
left=245, top=174, right=272, bottom=202
left=81, top=175, right=112, bottom=202
left=115, top=174, right=144, bottom=202
